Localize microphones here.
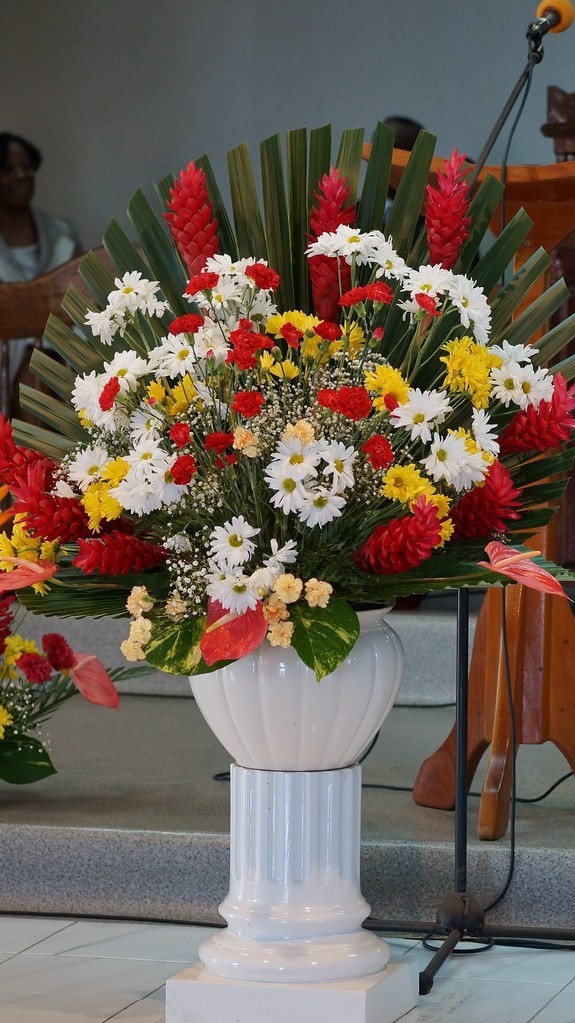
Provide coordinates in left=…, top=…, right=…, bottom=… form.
left=526, top=0, right=575, bottom=41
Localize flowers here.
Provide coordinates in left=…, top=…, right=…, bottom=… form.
left=0, top=120, right=575, bottom=784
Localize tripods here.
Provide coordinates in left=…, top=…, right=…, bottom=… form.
left=365, top=36, right=575, bottom=998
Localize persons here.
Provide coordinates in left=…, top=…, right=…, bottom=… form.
left=1, top=129, right=82, bottom=430
left=355, top=114, right=431, bottom=254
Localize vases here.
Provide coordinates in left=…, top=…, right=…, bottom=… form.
left=186, top=601, right=404, bottom=772
